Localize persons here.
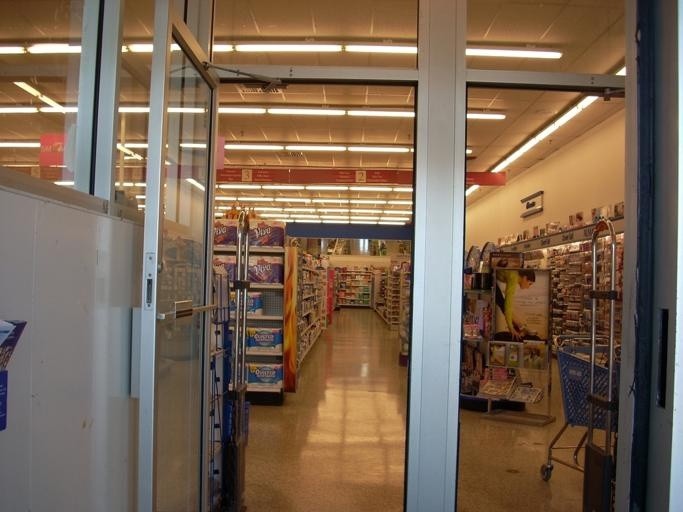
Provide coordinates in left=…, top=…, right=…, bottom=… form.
left=496, top=269, right=536, bottom=340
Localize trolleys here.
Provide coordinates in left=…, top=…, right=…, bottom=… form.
left=538, top=333, right=620, bottom=481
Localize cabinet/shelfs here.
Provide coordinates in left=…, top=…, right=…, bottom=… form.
left=460, top=239, right=623, bottom=412
left=209, top=217, right=335, bottom=404
left=339, top=268, right=371, bottom=308
left=375, top=261, right=413, bottom=366
